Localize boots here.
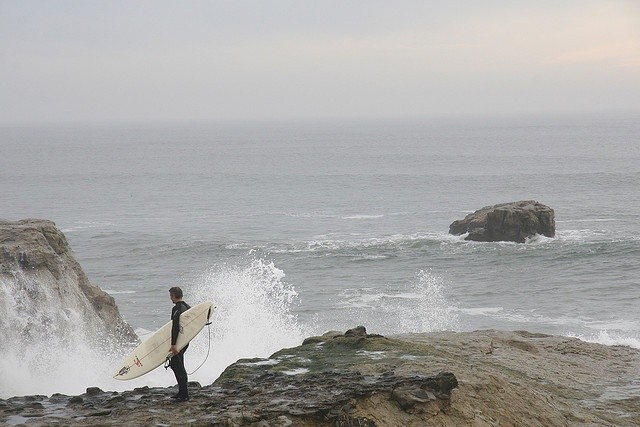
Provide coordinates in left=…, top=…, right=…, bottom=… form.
left=170, top=388, right=180, bottom=398
left=175, top=389, right=190, bottom=402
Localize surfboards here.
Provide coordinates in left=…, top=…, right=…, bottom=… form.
left=112, top=301, right=215, bottom=380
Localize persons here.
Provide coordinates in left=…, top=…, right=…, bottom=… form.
left=169, top=286, right=191, bottom=402
